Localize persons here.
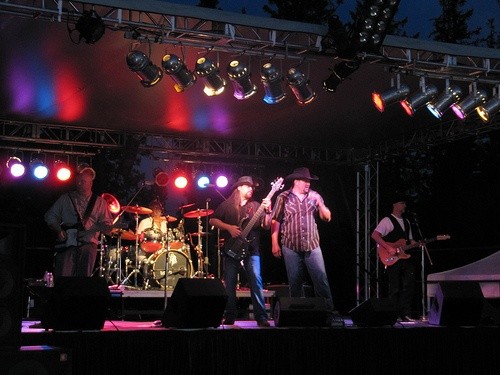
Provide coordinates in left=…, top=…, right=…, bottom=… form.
left=271, top=167, right=335, bottom=311
left=209, top=176, right=272, bottom=327
left=370, top=196, right=423, bottom=326
left=45, top=166, right=123, bottom=277
left=136, top=200, right=177, bottom=259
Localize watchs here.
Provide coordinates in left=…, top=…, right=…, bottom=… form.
left=264, top=210, right=272, bottom=214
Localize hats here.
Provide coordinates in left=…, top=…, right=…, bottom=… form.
left=75, top=162, right=95, bottom=179
left=386, top=188, right=418, bottom=201
left=230, top=175, right=259, bottom=190
left=286, top=168, right=319, bottom=180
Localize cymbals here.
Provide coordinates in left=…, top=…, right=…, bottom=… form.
left=192, top=232, right=209, bottom=236
left=121, top=205, right=153, bottom=214
left=169, top=203, right=194, bottom=212
left=158, top=217, right=177, bottom=221
left=184, top=209, right=214, bottom=218
left=105, top=228, right=135, bottom=240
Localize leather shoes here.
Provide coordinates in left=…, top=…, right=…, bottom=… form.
left=397, top=315, right=416, bottom=322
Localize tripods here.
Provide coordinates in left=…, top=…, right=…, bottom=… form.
left=92, top=213, right=154, bottom=292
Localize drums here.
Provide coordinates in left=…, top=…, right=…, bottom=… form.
left=140, top=227, right=164, bottom=253
left=119, top=245, right=141, bottom=270
left=165, top=228, right=184, bottom=249
left=143, top=248, right=194, bottom=289
left=106, top=246, right=123, bottom=270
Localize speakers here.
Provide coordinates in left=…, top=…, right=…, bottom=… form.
left=349, top=296, right=405, bottom=328
left=160, top=277, right=228, bottom=328
left=273, top=298, right=337, bottom=328
left=0, top=345, right=73, bottom=375
left=40, top=276, right=107, bottom=331
left=0, top=223, right=26, bottom=349
left=428, top=280, right=485, bottom=326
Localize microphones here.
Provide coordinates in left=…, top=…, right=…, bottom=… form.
left=144, top=181, right=155, bottom=185
left=204, top=184, right=216, bottom=187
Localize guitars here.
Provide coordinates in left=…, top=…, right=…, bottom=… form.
left=377, top=234, right=451, bottom=267
left=222, top=176, right=285, bottom=260
left=49, top=220, right=131, bottom=257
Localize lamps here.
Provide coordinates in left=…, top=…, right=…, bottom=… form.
left=190, top=170, right=209, bottom=189
left=210, top=169, right=228, bottom=187
left=153, top=168, right=172, bottom=185
left=64, top=0, right=500, bottom=123
left=6, top=156, right=25, bottom=178
left=26, top=159, right=48, bottom=180
left=170, top=170, right=188, bottom=189
left=52, top=160, right=70, bottom=182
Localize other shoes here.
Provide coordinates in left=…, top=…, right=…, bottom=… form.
left=257, top=319, right=271, bottom=327
left=220, top=320, right=234, bottom=324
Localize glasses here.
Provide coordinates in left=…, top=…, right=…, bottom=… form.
left=153, top=207, right=160, bottom=210
